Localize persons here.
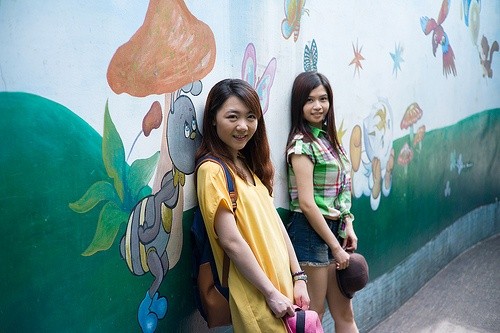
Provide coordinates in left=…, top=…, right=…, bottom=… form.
left=194, top=78, right=310, bottom=333
left=285, top=72, right=360, bottom=333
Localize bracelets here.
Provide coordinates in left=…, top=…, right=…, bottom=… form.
left=292, top=270, right=308, bottom=284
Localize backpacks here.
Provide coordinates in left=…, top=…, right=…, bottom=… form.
left=190, top=155, right=237, bottom=329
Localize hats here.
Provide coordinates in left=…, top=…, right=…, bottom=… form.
left=336, top=249, right=368, bottom=299
left=283, top=305, right=324, bottom=333
